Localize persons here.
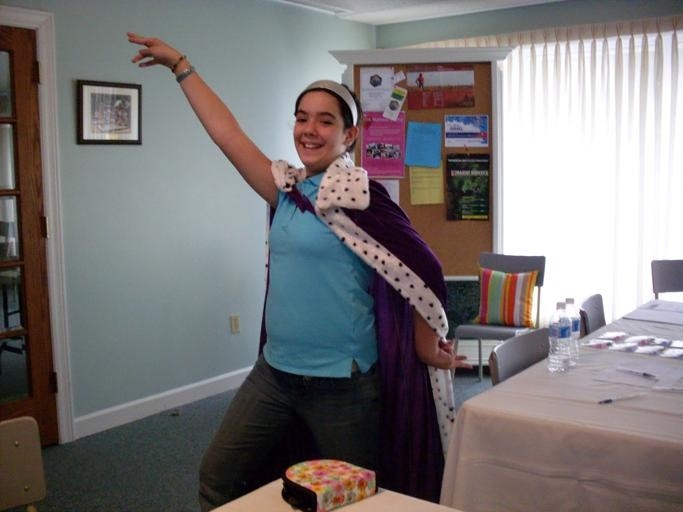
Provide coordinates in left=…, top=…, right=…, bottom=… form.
left=366, top=149, right=371, bottom=159
left=106, top=107, right=128, bottom=126
left=377, top=144, right=387, bottom=159
left=415, top=72, right=425, bottom=91
left=126, top=32, right=474, bottom=512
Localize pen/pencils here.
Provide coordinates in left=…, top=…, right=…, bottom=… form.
left=599, top=393, right=648, bottom=405
left=616, top=366, right=656, bottom=377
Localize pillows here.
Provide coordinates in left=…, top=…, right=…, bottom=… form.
left=470, top=262, right=540, bottom=327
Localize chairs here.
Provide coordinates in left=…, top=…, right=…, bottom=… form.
left=0, top=415, right=51, bottom=511
left=650, top=259, right=682, bottom=302
left=488, top=325, right=548, bottom=386
left=449, top=249, right=548, bottom=381
left=577, top=293, right=606, bottom=337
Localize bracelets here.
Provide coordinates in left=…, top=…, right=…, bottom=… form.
left=176, top=67, right=194, bottom=83
left=172, top=54, right=186, bottom=72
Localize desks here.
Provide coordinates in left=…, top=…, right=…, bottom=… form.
left=204, top=461, right=462, bottom=512
left=440, top=297, right=682, bottom=511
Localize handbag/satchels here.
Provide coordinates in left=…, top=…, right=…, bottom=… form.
left=282, top=459, right=378, bottom=512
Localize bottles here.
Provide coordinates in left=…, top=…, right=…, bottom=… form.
left=561, top=297, right=582, bottom=365
left=549, top=302, right=570, bottom=372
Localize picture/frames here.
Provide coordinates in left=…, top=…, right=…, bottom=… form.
left=72, top=77, right=143, bottom=147
